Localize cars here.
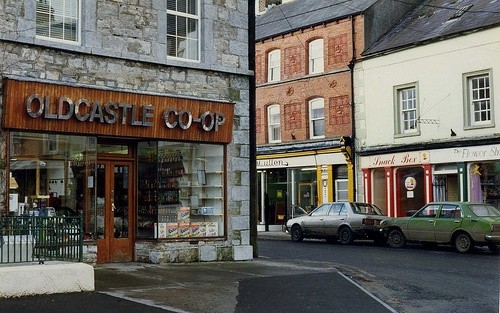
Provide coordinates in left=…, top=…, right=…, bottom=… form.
left=286, top=201, right=389, bottom=245
left=380, top=202, right=500, bottom=254
